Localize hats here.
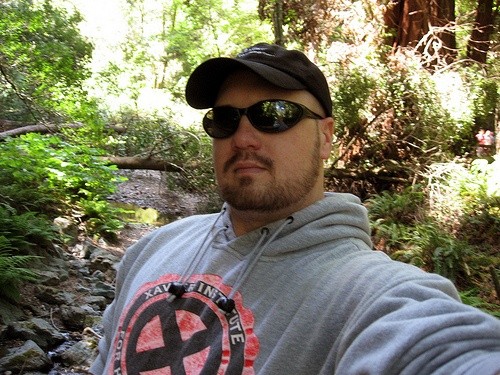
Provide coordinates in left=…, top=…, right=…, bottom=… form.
left=185, top=42, right=332, bottom=117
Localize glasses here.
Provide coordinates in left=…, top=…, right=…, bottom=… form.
left=203, top=99, right=324, bottom=139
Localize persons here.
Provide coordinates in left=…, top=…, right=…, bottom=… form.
left=90, top=42, right=500, bottom=375
